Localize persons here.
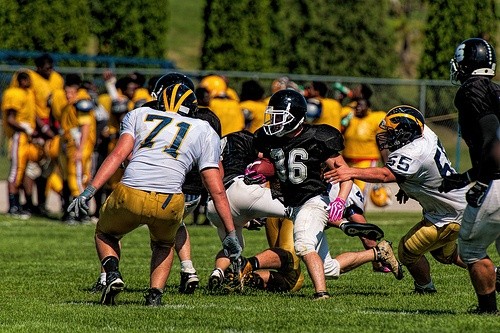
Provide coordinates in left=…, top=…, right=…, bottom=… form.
left=323, top=104, right=500, bottom=295
left=183, top=75, right=387, bottom=225
left=1, top=53, right=153, bottom=224
left=93, top=72, right=224, bottom=293
left=449, top=38, right=500, bottom=315
left=68, top=84, right=242, bottom=306
left=211, top=90, right=405, bottom=299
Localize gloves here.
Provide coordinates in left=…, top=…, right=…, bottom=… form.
left=67, top=186, right=97, bottom=218
left=443, top=172, right=472, bottom=192
left=466, top=181, right=489, bottom=208
left=244, top=164, right=266, bottom=184
left=222, top=231, right=242, bottom=260
left=326, top=198, right=347, bottom=222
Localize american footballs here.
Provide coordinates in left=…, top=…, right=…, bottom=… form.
left=250, top=158, right=276, bottom=179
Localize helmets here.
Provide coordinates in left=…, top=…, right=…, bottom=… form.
left=454, top=37, right=496, bottom=84
left=200, top=75, right=227, bottom=97
left=159, top=83, right=198, bottom=118
left=382, top=104, right=425, bottom=146
left=152, top=72, right=195, bottom=100
left=268, top=90, right=308, bottom=137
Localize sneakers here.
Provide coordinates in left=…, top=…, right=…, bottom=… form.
left=411, top=281, right=437, bottom=295
left=88, top=261, right=224, bottom=307
left=373, top=261, right=391, bottom=273
left=374, top=239, right=404, bottom=280
left=340, top=222, right=384, bottom=241
left=313, top=292, right=329, bottom=301
left=233, top=256, right=253, bottom=295
